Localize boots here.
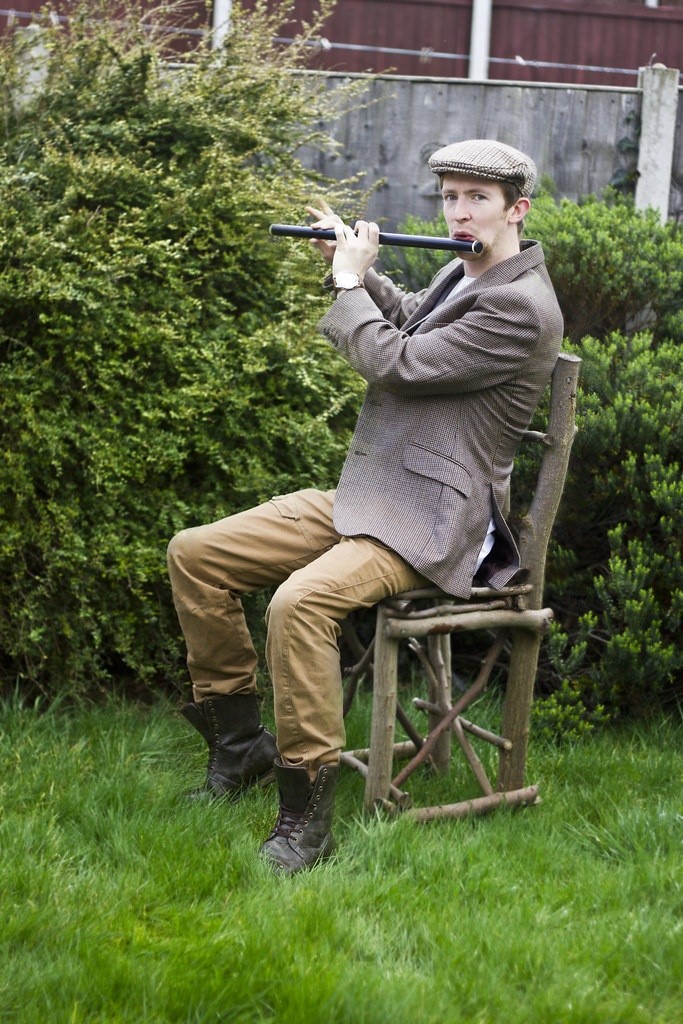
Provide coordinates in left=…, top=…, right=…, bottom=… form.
left=259, top=758, right=338, bottom=878
left=174, top=693, right=282, bottom=811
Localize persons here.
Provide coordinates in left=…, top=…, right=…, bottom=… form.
left=167, top=140, right=566, bottom=875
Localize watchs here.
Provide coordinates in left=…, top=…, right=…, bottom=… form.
left=333, top=271, right=365, bottom=293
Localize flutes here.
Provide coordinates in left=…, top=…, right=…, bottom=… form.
left=266, top=222, right=484, bottom=255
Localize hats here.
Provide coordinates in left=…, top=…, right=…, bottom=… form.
left=427, top=139, right=536, bottom=199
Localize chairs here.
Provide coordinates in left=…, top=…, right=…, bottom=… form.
left=338, top=351, right=581, bottom=823
